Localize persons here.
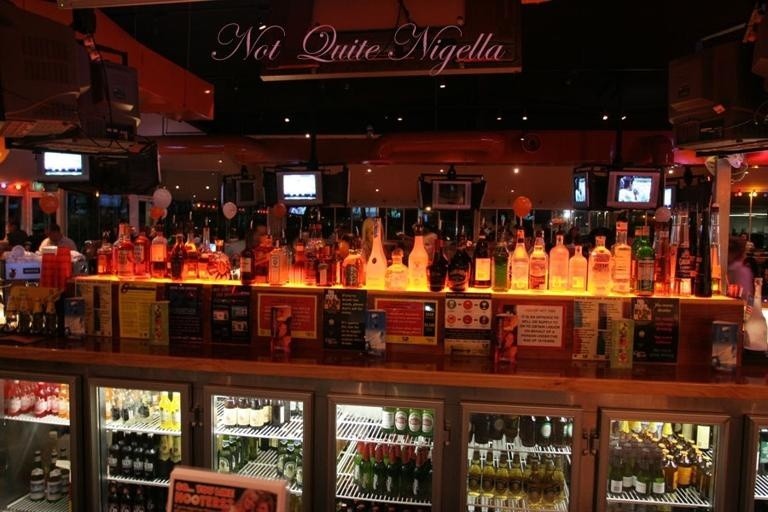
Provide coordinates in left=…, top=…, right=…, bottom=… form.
left=39, top=224, right=77, bottom=251
left=728, top=237, right=753, bottom=301
left=617, top=176, right=641, bottom=202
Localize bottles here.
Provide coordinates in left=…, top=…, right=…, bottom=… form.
left=636, top=225, right=656, bottom=296
left=511, top=226, right=530, bottom=291
left=121, top=431, right=133, bottom=476
left=688, top=212, right=698, bottom=296
left=632, top=443, right=645, bottom=489
left=411, top=447, right=428, bottom=500
left=239, top=227, right=256, bottom=285
left=448, top=234, right=472, bottom=292
left=306, top=207, right=325, bottom=286
left=669, top=213, right=681, bottom=295
left=653, top=221, right=670, bottom=297
left=296, top=448, right=304, bottom=488
left=691, top=450, right=703, bottom=487
left=59, top=384, right=68, bottom=418
left=31, top=296, right=44, bottom=335
left=663, top=454, right=678, bottom=493
left=171, top=392, right=181, bottom=430
left=224, top=396, right=237, bottom=428
left=554, top=455, right=565, bottom=503
left=46, top=448, right=62, bottom=503
left=51, top=386, right=60, bottom=415
left=683, top=439, right=695, bottom=451
left=608, top=446, right=623, bottom=494
left=144, top=433, right=157, bottom=480
left=696, top=424, right=712, bottom=451
left=45, top=296, right=57, bottom=334
left=427, top=238, right=448, bottom=291
left=636, top=447, right=652, bottom=496
left=342, top=243, right=362, bottom=287
left=250, top=398, right=264, bottom=429
left=757, top=428, right=768, bottom=476
left=184, top=222, right=198, bottom=280
left=132, top=485, right=146, bottom=512
left=550, top=416, right=568, bottom=447
left=523, top=454, right=533, bottom=499
left=29, top=450, right=46, bottom=501
left=117, top=431, right=125, bottom=447
left=198, top=225, right=213, bottom=279
left=96, top=231, right=114, bottom=275
left=171, top=436, right=182, bottom=472
left=569, top=242, right=588, bottom=293
left=421, top=409, right=435, bottom=437
left=469, top=227, right=495, bottom=288
left=385, top=247, right=408, bottom=290
left=277, top=440, right=287, bottom=476
left=407, top=215, right=428, bottom=291
left=150, top=224, right=170, bottom=277
left=45, top=384, right=52, bottom=414
left=474, top=413, right=491, bottom=444
left=394, top=407, right=409, bottom=435
left=145, top=486, right=157, bottom=512
left=263, top=398, right=270, bottom=425
left=113, top=222, right=134, bottom=277
left=273, top=399, right=285, bottom=425
left=696, top=455, right=710, bottom=492
left=674, top=445, right=684, bottom=461
left=267, top=239, right=288, bottom=286
left=104, top=387, right=159, bottom=423
left=526, top=459, right=542, bottom=506
left=480, top=450, right=495, bottom=499
left=536, top=416, right=553, bottom=446
left=107, top=429, right=121, bottom=475
left=677, top=450, right=693, bottom=487
left=4, top=379, right=12, bottom=415
left=520, top=415, right=537, bottom=446
left=59, top=447, right=70, bottom=495
left=631, top=225, right=642, bottom=294
left=399, top=446, right=413, bottom=498
left=159, top=391, right=172, bottom=429
left=467, top=449, right=482, bottom=498
left=20, top=381, right=31, bottom=414
left=170, top=234, right=186, bottom=280
left=133, top=432, right=144, bottom=477
left=491, top=234, right=511, bottom=292
left=673, top=216, right=692, bottom=296
left=700, top=461, right=713, bottom=499
left=283, top=440, right=296, bottom=482
left=18, top=295, right=30, bottom=335
left=632, top=421, right=644, bottom=437
left=648, top=422, right=659, bottom=439
left=542, top=455, right=556, bottom=506
left=381, top=406, right=395, bottom=433
left=158, top=435, right=171, bottom=478
left=610, top=220, right=632, bottom=294
left=528, top=229, right=549, bottom=291
left=503, top=415, right=519, bottom=444
left=207, top=238, right=232, bottom=280
left=359, top=446, right=372, bottom=494
left=134, top=222, right=152, bottom=278
left=621, top=442, right=633, bottom=491
left=8, top=379, right=22, bottom=416
left=217, top=434, right=260, bottom=475
left=743, top=276, right=768, bottom=368
left=365, top=217, right=388, bottom=289
left=694, top=211, right=713, bottom=297
left=5, top=296, right=18, bottom=332
left=130, top=432, right=138, bottom=449
left=549, top=232, right=570, bottom=292
left=291, top=241, right=307, bottom=286
left=651, top=449, right=666, bottom=496
left=108, top=480, right=120, bottom=512
left=120, top=484, right=132, bottom=512
left=495, top=451, right=510, bottom=500
left=28, top=381, right=36, bottom=410
left=490, top=415, right=505, bottom=440
left=385, top=446, right=399, bottom=497
left=669, top=440, right=678, bottom=453
left=332, top=250, right=344, bottom=286
left=509, top=453, right=522, bottom=499
left=372, top=445, right=386, bottom=495
left=353, top=441, right=365, bottom=484
left=34, top=382, right=47, bottom=417
left=677, top=433, right=686, bottom=446
left=237, top=397, right=250, bottom=428
left=657, top=434, right=671, bottom=450
left=407, top=408, right=422, bottom=436
left=588, top=235, right=613, bottom=293
left=688, top=444, right=698, bottom=460
left=315, top=253, right=333, bottom=286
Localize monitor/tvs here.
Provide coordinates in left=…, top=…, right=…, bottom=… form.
left=288, top=206, right=307, bottom=216
left=275, top=171, right=323, bottom=205
left=328, top=168, right=352, bottom=207
left=475, top=181, right=487, bottom=210
left=432, top=180, right=472, bottom=209
left=663, top=186, right=675, bottom=207
left=666, top=35, right=768, bottom=149
left=571, top=172, right=594, bottom=209
left=234, top=179, right=256, bottom=206
left=36, top=151, right=89, bottom=181
left=607, top=171, right=660, bottom=209
left=417, top=180, right=429, bottom=209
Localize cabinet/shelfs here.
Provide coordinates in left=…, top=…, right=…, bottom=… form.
left=739, top=412, right=768, bottom=512
left=594, top=408, right=730, bottom=512
left=86, top=376, right=194, bottom=512
left=455, top=399, right=586, bottom=512
left=201, top=384, right=314, bottom=512
left=0, top=368, right=82, bottom=512
left=324, top=393, right=447, bottom=512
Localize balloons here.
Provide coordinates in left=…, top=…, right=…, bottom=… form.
left=153, top=189, right=171, bottom=209
left=222, top=202, right=237, bottom=219
left=513, top=196, right=533, bottom=218
left=655, top=207, right=671, bottom=222
left=39, top=192, right=57, bottom=215
left=274, top=203, right=287, bottom=217
